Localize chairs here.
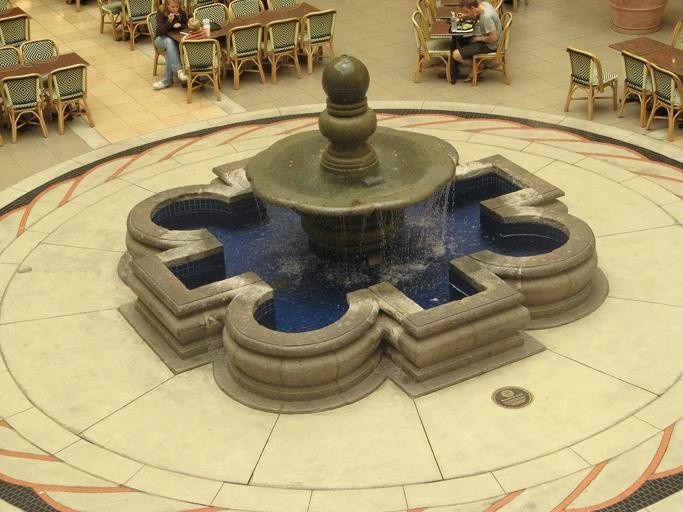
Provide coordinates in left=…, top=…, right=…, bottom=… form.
left=97, top=0, right=336, bottom=104
left=563, top=20, right=682, bottom=141
left=410, top=0, right=512, bottom=86
left=0, top=14, right=94, bottom=144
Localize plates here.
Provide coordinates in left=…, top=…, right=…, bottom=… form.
left=451, top=26, right=473, bottom=33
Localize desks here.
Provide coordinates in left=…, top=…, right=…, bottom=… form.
left=0, top=6, right=32, bottom=19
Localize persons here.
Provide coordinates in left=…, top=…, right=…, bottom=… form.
left=151, top=1, right=188, bottom=90
left=179, top=18, right=206, bottom=41
left=451, top=0, right=501, bottom=82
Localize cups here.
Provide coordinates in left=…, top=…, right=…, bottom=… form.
left=203, top=19, right=210, bottom=25
left=204, top=25, right=211, bottom=37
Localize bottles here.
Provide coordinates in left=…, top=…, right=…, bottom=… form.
left=451, top=13, right=457, bottom=31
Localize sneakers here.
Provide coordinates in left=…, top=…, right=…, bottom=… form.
left=469, top=63, right=487, bottom=78
left=463, top=70, right=484, bottom=83
left=152, top=80, right=167, bottom=90
left=177, top=68, right=187, bottom=81
